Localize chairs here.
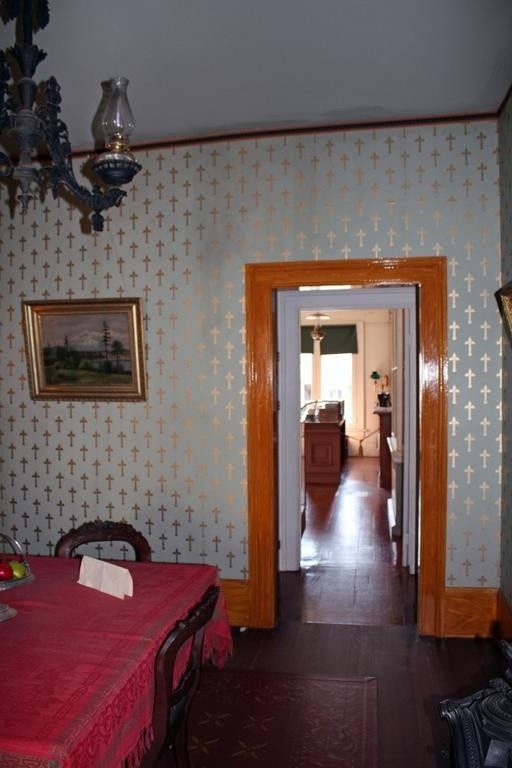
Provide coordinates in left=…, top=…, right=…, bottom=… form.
left=128, top=584, right=220, bottom=768
left=54, top=520, right=152, bottom=563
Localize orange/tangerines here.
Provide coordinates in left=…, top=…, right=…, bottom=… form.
left=10, top=560, right=27, bottom=579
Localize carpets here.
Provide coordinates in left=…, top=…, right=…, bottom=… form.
left=169, top=665, right=378, bottom=768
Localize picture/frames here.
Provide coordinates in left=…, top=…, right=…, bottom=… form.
left=20, top=298, right=148, bottom=402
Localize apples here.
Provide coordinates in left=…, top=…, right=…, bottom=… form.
left=0, top=562, right=14, bottom=582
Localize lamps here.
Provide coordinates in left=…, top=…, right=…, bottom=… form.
left=306, top=311, right=331, bottom=344
left=0, top=0, right=142, bottom=235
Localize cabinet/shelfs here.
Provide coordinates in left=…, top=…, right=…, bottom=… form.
left=304, top=419, right=345, bottom=485
left=373, top=407, right=391, bottom=490
